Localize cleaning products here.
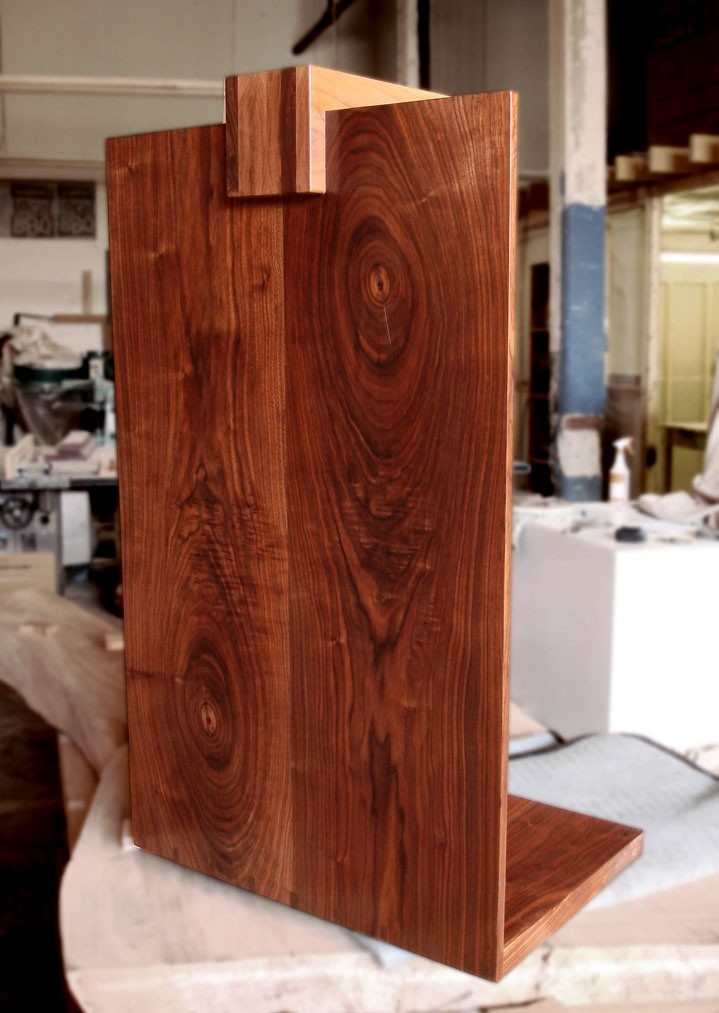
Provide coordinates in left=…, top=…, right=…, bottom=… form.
left=609, top=436, right=637, bottom=504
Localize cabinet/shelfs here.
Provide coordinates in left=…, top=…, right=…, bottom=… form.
left=522, top=260, right=552, bottom=496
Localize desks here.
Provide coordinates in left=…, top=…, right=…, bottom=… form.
left=509, top=501, right=719, bottom=749
left=1, top=551, right=719, bottom=1012
left=660, top=421, right=707, bottom=491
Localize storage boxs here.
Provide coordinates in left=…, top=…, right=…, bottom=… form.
left=0, top=492, right=101, bottom=608
